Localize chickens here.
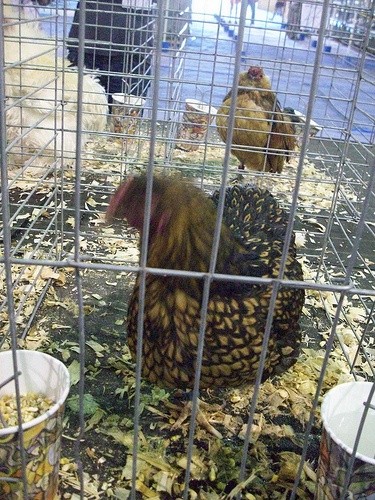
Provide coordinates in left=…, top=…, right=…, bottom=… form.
left=106, top=171, right=304, bottom=441
left=216, top=66, right=319, bottom=175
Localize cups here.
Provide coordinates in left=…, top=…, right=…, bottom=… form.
left=0, top=349, right=72, bottom=500
left=110, top=92, right=147, bottom=145
left=315, top=380, right=375, bottom=500
left=294, top=108, right=322, bottom=158
left=176, top=98, right=218, bottom=153
